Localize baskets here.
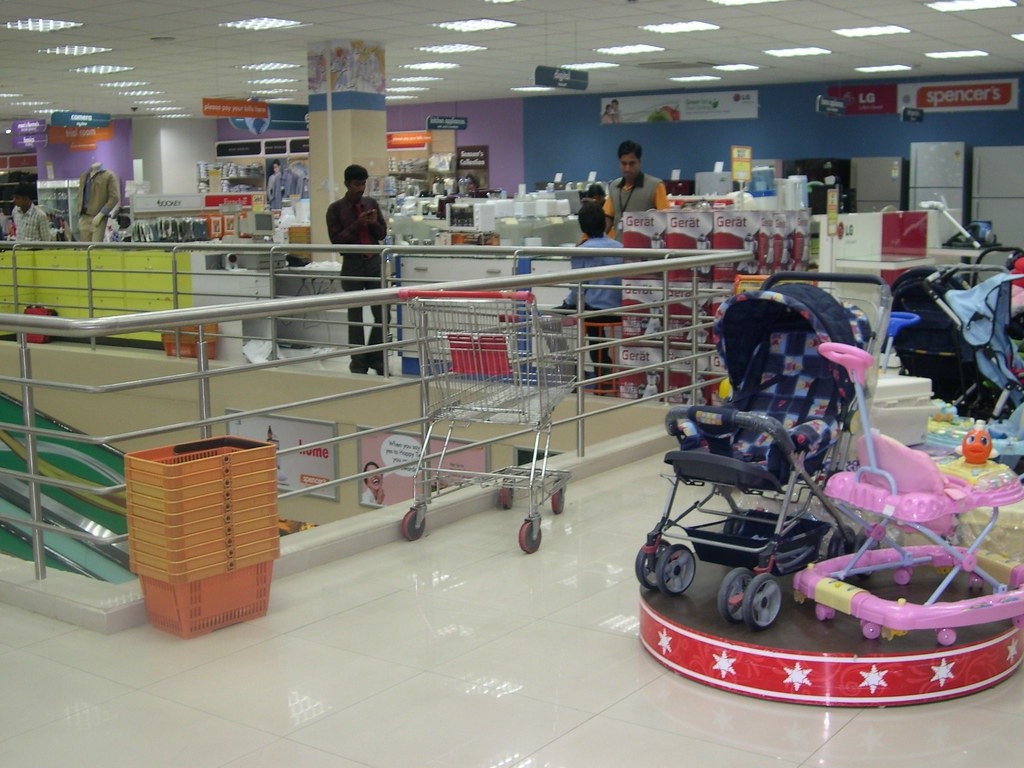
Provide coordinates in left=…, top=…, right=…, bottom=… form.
left=125, top=434, right=282, bottom=639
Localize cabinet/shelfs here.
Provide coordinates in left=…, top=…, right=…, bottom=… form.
left=0, top=129, right=597, bottom=393
left=812, top=210, right=998, bottom=367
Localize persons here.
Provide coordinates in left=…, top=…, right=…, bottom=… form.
left=77, top=163, right=118, bottom=240
left=326, top=165, right=392, bottom=376
left=579, top=141, right=669, bottom=245
left=10, top=183, right=51, bottom=241
left=362, top=463, right=384, bottom=505
left=611, top=99, right=621, bottom=124
left=268, top=160, right=281, bottom=203
left=601, top=105, right=612, bottom=124
left=540, top=205, right=624, bottom=396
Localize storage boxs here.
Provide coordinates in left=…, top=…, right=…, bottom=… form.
left=621, top=209, right=811, bottom=410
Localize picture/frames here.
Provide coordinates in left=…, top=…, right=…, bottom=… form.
left=513, top=446, right=567, bottom=468
left=225, top=409, right=340, bottom=503
left=356, top=424, right=493, bottom=509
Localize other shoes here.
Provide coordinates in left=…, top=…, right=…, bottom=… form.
left=349, top=359, right=369, bottom=374
left=593, top=375, right=621, bottom=396
left=363, top=346, right=388, bottom=374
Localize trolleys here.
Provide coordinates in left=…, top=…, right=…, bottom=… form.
left=396, top=285, right=579, bottom=554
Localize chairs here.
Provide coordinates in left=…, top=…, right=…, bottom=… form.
left=583, top=322, right=622, bottom=395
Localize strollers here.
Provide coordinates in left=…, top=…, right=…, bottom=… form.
left=890, top=244, right=1024, bottom=424
left=634, top=269, right=894, bottom=633
